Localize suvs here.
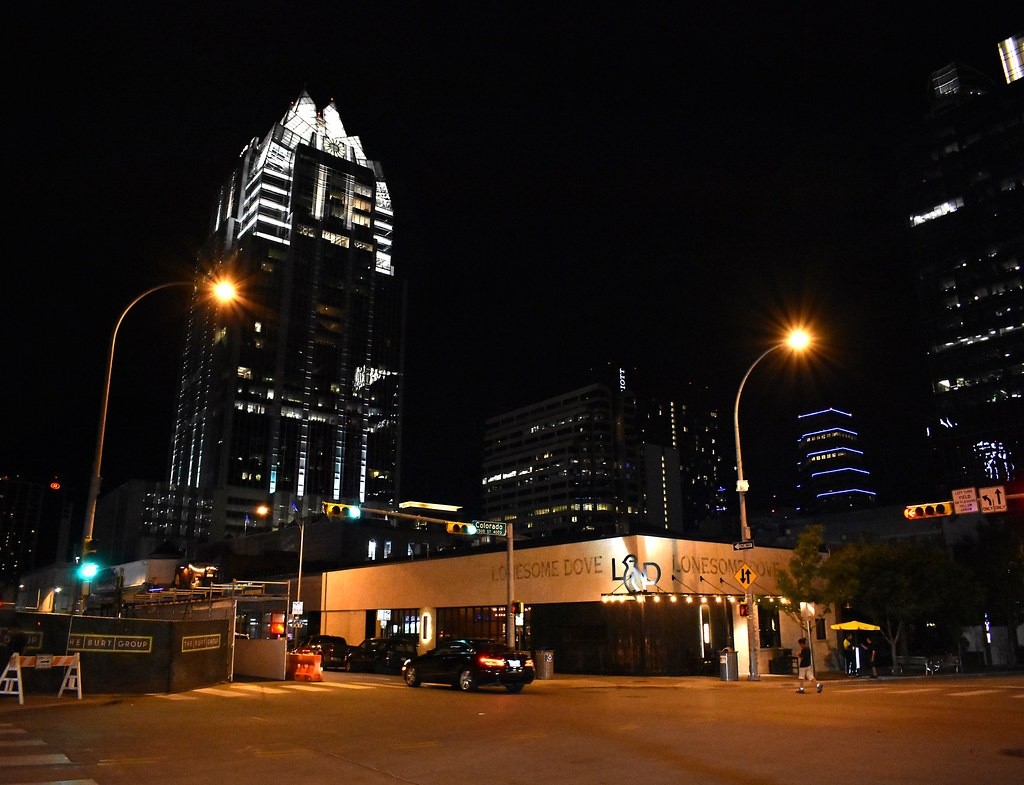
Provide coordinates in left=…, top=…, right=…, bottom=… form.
left=305, top=636, right=350, bottom=670
left=349, top=638, right=418, bottom=674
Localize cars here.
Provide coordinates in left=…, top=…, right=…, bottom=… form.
left=400, top=638, right=537, bottom=693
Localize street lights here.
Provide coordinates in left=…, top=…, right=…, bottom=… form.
left=255, top=505, right=305, bottom=650
left=78, top=275, right=237, bottom=608
left=732, top=325, right=818, bottom=679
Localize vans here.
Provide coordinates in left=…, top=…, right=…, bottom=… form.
left=888, top=656, right=940, bottom=675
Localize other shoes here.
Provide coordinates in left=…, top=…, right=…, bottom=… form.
left=817, top=684, right=823, bottom=693
left=869, top=675, right=878, bottom=679
left=796, top=688, right=805, bottom=693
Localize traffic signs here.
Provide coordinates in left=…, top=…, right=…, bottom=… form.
left=732, top=542, right=754, bottom=551
left=977, top=486, right=1008, bottom=514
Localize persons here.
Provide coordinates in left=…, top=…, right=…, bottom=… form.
left=843, top=632, right=855, bottom=675
left=861, top=636, right=880, bottom=679
left=794, top=637, right=824, bottom=694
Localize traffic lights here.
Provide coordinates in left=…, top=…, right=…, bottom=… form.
left=323, top=503, right=360, bottom=522
left=447, top=521, right=477, bottom=536
left=738, top=602, right=750, bottom=617
left=81, top=536, right=99, bottom=586
left=512, top=601, right=523, bottom=615
left=903, top=502, right=954, bottom=521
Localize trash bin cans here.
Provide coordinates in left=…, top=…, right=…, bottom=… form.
left=534, top=649, right=555, bottom=679
left=719, top=647, right=739, bottom=681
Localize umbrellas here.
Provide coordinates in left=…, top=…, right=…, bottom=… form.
left=829, top=620, right=883, bottom=634
